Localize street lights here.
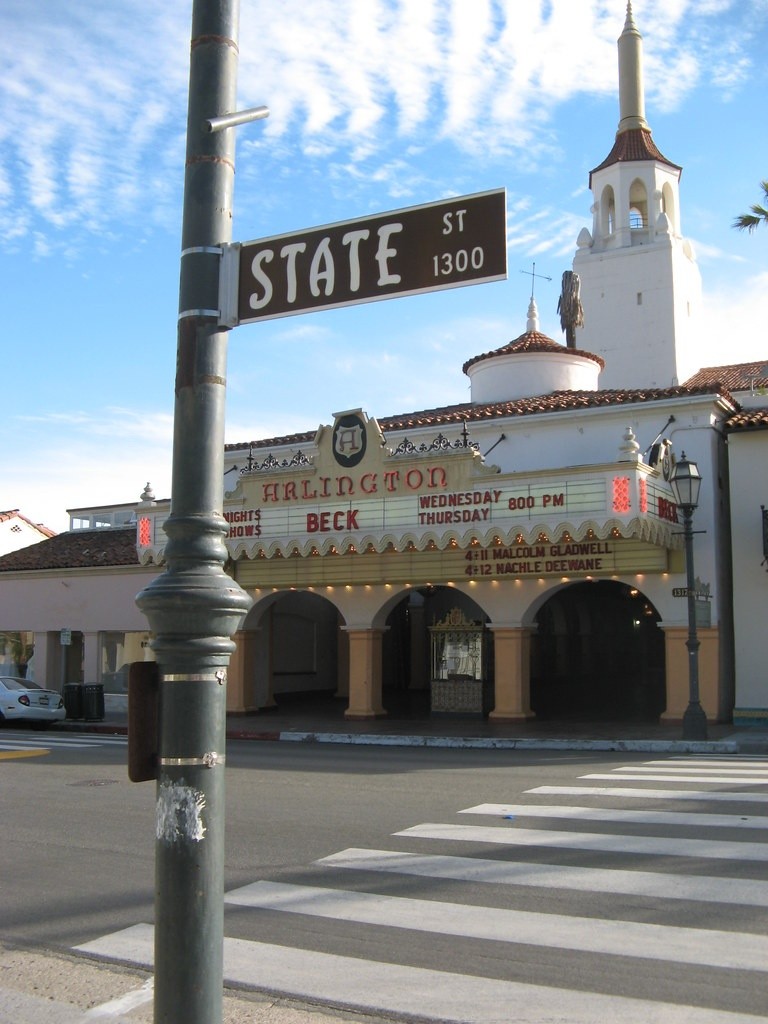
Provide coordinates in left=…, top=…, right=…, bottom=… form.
left=667, top=450, right=708, bottom=739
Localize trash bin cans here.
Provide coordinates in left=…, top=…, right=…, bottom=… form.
left=63, top=682, right=82, bottom=721
left=82, top=683, right=105, bottom=722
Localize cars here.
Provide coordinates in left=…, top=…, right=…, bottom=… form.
left=0, top=676, right=66, bottom=731
